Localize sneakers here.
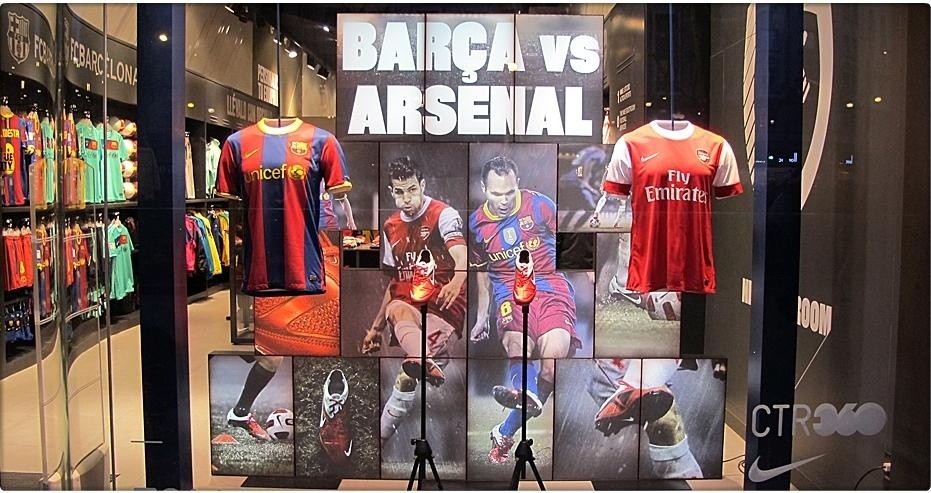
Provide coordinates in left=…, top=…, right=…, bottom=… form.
left=592, top=210, right=599, bottom=219
left=318, top=367, right=355, bottom=466
left=487, top=422, right=516, bottom=467
left=592, top=385, right=674, bottom=438
left=512, top=248, right=538, bottom=305
left=491, top=384, right=543, bottom=418
left=402, top=356, right=446, bottom=387
left=607, top=274, right=647, bottom=312
left=614, top=222, right=619, bottom=228
left=408, top=247, right=439, bottom=304
left=226, top=407, right=273, bottom=442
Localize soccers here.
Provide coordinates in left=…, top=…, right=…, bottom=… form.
left=646, top=291, right=680, bottom=321
left=110, top=115, right=139, bottom=200
left=266, top=408, right=294, bottom=442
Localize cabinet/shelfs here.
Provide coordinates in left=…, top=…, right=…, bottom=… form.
left=0, top=2, right=278, bottom=392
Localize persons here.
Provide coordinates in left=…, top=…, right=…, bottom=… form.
left=227, top=357, right=283, bottom=443
left=588, top=358, right=705, bottom=479
left=468, top=157, right=578, bottom=465
left=360, top=157, right=468, bottom=449
left=557, top=142, right=609, bottom=231
left=608, top=233, right=647, bottom=310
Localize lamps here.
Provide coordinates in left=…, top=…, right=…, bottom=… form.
left=224, top=1, right=331, bottom=81
